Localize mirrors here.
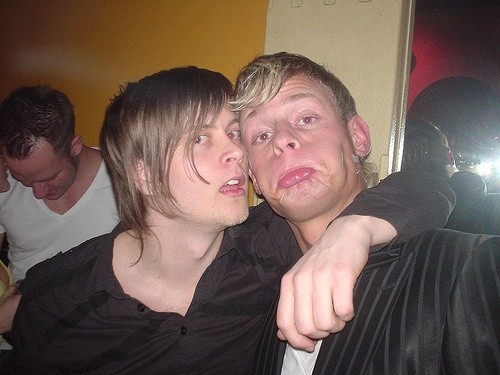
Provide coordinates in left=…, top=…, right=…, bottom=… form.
left=387, top=0, right=500, bottom=236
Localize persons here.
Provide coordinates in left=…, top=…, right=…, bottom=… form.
left=0, top=86, right=121, bottom=366
left=230, top=48, right=500, bottom=374
left=399, top=118, right=500, bottom=237
left=0, top=64, right=456, bottom=374
